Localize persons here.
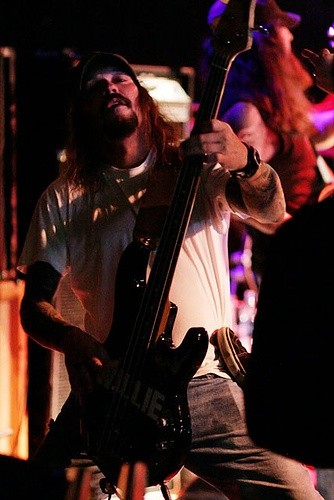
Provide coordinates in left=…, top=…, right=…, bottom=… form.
left=19, top=51, right=324, bottom=500
left=211, top=0, right=334, bottom=469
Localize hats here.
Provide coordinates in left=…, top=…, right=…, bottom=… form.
left=77, top=51, right=137, bottom=100
left=206, top=0, right=301, bottom=31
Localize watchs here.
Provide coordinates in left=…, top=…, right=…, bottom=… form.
left=230, top=141, right=262, bottom=178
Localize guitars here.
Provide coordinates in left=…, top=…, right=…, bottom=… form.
left=80, top=0, right=270, bottom=490
